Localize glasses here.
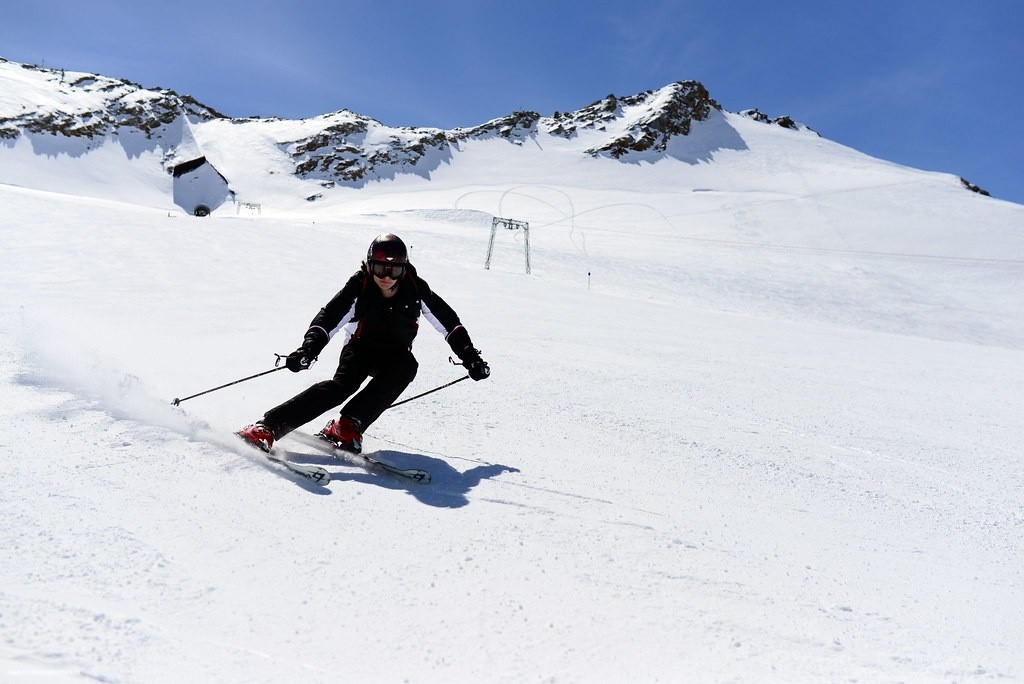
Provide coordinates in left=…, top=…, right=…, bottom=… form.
left=368, top=264, right=405, bottom=280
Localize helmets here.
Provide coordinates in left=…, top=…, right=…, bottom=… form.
left=367, top=235, right=407, bottom=263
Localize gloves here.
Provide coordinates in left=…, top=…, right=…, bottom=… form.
left=445, top=325, right=490, bottom=381
left=286, top=325, right=330, bottom=373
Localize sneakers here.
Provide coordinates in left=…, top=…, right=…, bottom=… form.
left=314, top=420, right=364, bottom=454
left=237, top=416, right=274, bottom=454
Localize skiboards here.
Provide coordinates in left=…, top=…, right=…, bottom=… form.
left=262, top=430, right=432, bottom=488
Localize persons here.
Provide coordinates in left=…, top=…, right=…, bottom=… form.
left=239, top=233, right=491, bottom=453
